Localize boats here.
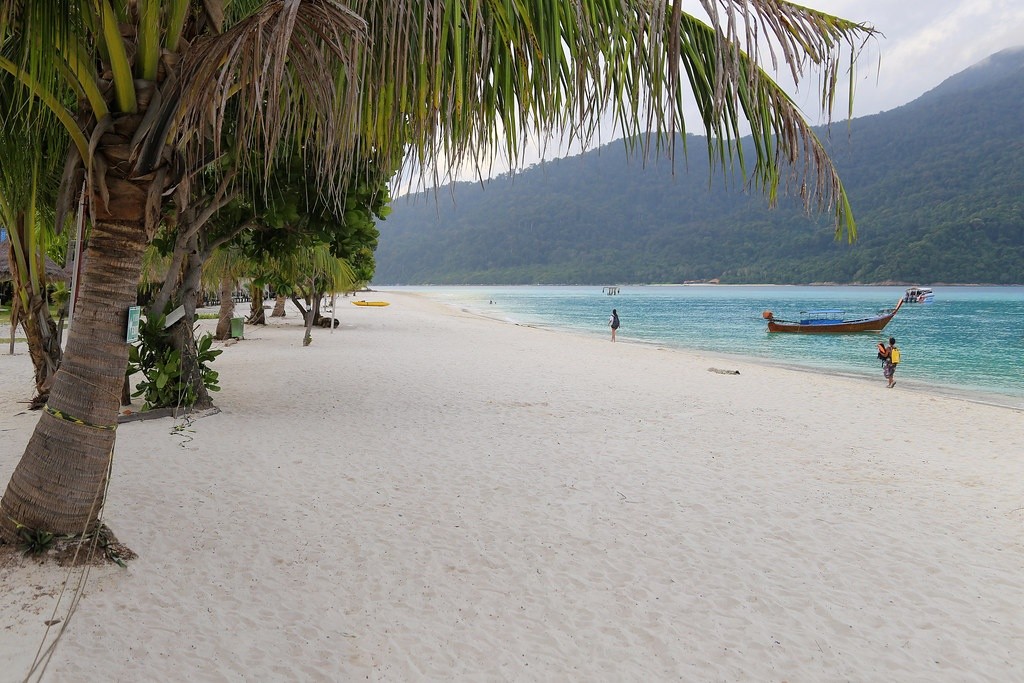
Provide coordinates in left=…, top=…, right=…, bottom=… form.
left=762, top=298, right=905, bottom=333
left=903, top=286, right=935, bottom=303
left=353, top=301, right=390, bottom=307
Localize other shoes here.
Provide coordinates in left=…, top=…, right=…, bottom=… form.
left=886, top=382, right=896, bottom=388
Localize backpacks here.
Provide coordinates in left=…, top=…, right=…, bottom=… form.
left=612, top=315, right=619, bottom=328
left=888, top=347, right=900, bottom=364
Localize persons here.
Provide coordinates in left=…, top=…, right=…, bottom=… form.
left=880, top=338, right=899, bottom=388
left=608, top=309, right=620, bottom=342
